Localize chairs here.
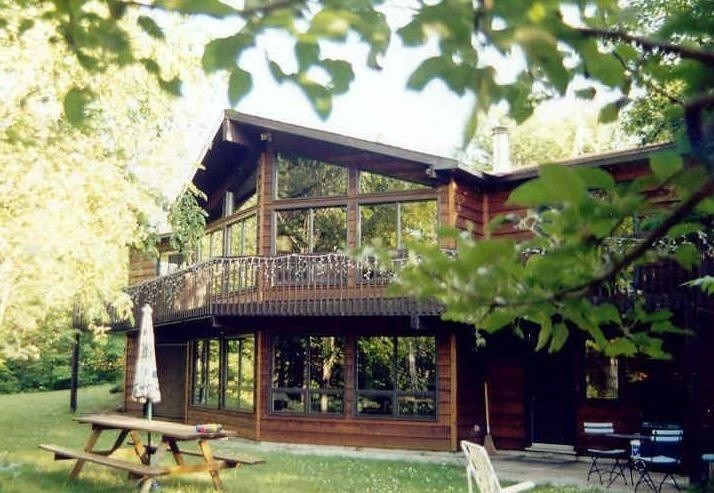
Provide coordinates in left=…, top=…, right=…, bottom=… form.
left=583, top=422, right=683, bottom=493
left=461, top=441, right=534, bottom=493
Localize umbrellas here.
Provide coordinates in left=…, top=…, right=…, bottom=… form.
left=135, top=299, right=167, bottom=479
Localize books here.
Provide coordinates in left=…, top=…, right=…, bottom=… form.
left=197, top=420, right=224, bottom=432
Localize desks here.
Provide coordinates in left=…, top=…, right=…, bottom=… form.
left=68, top=415, right=238, bottom=493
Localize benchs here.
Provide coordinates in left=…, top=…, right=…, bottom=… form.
left=39, top=444, right=171, bottom=480
left=127, top=441, right=266, bottom=467
